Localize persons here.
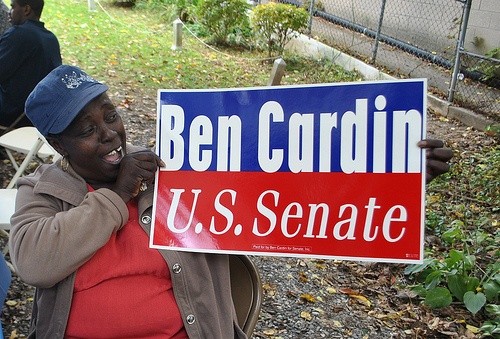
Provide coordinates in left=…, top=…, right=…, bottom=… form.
left=8, top=64, right=456, bottom=338
left=0, top=0, right=63, bottom=158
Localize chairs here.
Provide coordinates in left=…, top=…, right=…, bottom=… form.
left=0, top=126, right=62, bottom=190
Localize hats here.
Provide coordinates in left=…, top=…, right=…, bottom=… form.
left=24, top=64, right=109, bottom=138
